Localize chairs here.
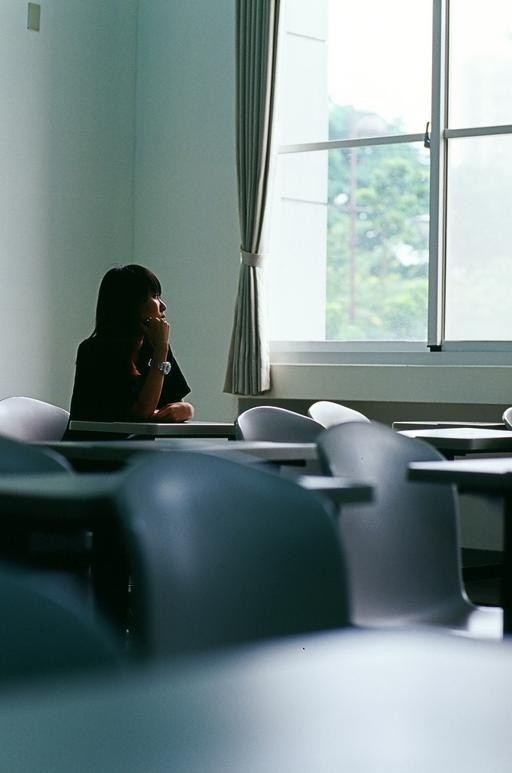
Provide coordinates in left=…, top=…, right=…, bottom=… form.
left=1, top=391, right=512, bottom=773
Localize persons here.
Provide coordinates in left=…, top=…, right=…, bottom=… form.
left=61, top=263, right=194, bottom=638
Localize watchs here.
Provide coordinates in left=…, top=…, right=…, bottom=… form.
left=147, top=357, right=171, bottom=376
